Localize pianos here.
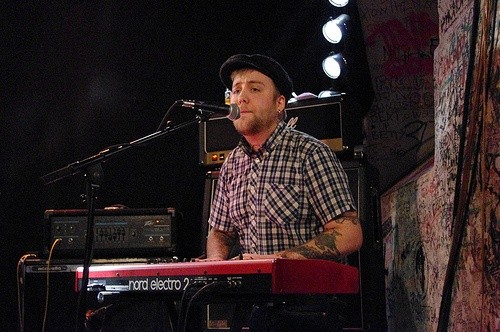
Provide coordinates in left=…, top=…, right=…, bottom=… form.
left=22, top=254, right=360, bottom=304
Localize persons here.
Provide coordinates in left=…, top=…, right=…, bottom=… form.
left=193, top=53, right=363, bottom=261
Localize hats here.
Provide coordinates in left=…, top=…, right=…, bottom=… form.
left=218, top=54, right=293, bottom=100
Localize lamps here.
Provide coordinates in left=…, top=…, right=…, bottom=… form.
left=323, top=54, right=346, bottom=79
left=322, top=15, right=350, bottom=43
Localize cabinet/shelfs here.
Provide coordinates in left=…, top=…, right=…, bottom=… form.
left=203, top=154, right=386, bottom=332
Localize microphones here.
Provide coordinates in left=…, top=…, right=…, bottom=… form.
left=176, top=99, right=241, bottom=121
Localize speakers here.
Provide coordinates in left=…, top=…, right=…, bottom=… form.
left=20, top=209, right=194, bottom=332
left=201, top=96, right=389, bottom=332
